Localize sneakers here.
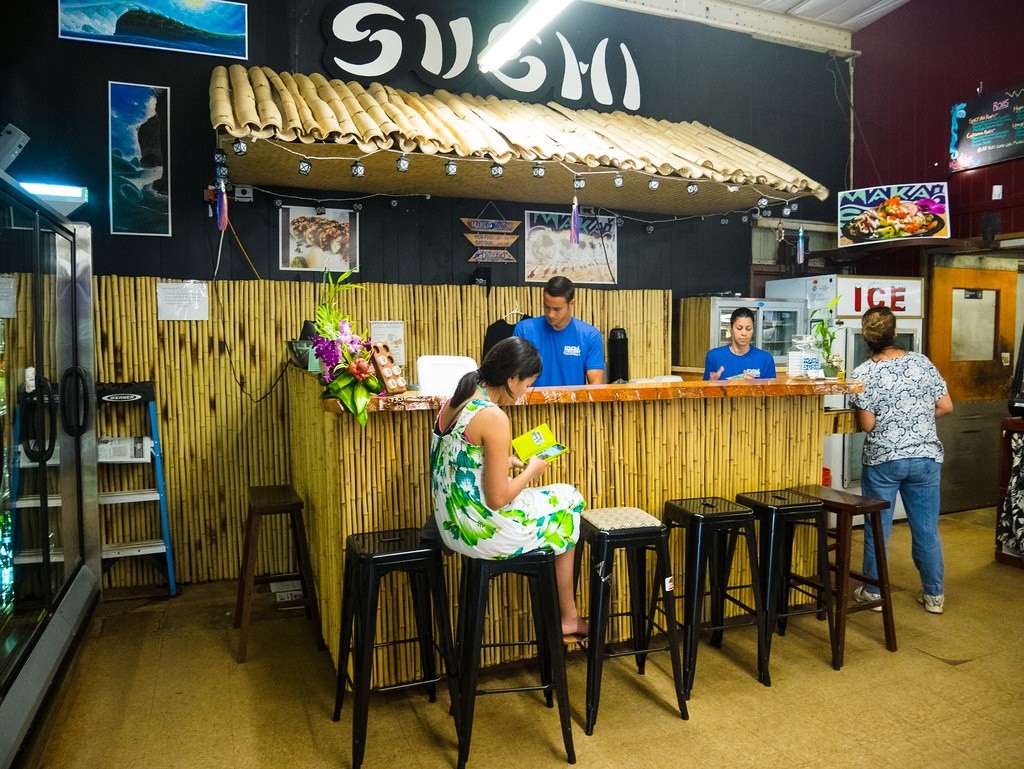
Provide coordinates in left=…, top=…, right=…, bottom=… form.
left=853, top=587, right=882, bottom=612
left=918, top=587, right=945, bottom=612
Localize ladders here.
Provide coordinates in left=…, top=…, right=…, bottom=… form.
left=8, top=380, right=64, bottom=610
left=94, top=381, right=177, bottom=600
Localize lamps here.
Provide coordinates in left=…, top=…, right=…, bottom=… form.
left=213, top=139, right=798, bottom=235
left=477, top=0, right=571, bottom=73
left=20, top=181, right=89, bottom=219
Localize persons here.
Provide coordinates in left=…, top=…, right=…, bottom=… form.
left=430, top=335, right=588, bottom=644
left=512, top=276, right=604, bottom=387
left=852, top=306, right=954, bottom=613
left=702, top=308, right=776, bottom=381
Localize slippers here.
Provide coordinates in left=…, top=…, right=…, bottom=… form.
left=562, top=620, right=589, bottom=643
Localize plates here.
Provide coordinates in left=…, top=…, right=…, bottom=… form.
left=842, top=212, right=945, bottom=241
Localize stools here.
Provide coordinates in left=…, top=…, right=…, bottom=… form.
left=233, top=484, right=325, bottom=667
left=331, top=483, right=898, bottom=769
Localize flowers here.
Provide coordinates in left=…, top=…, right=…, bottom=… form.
left=313, top=268, right=394, bottom=430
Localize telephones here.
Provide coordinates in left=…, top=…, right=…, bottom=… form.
left=300, top=319, right=318, bottom=340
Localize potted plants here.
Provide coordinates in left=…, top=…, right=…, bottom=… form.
left=808, top=295, right=840, bottom=376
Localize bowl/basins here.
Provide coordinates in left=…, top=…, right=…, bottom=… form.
left=285, top=340, right=315, bottom=370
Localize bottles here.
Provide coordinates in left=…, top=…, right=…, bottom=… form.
left=786, top=335, right=820, bottom=382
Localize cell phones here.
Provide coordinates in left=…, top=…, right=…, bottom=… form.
left=524, top=444, right=567, bottom=465
